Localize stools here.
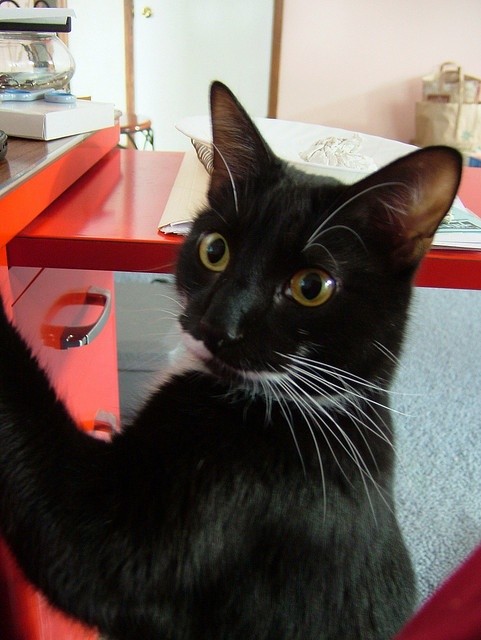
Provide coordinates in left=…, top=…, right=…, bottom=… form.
left=117, top=107, right=153, bottom=151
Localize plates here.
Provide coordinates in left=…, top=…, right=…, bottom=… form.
left=176, top=117, right=422, bottom=185
left=44, top=91, right=77, bottom=103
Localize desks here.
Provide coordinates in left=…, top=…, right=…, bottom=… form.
left=10, top=145, right=481, bottom=293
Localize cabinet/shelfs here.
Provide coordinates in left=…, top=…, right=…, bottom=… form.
left=1, top=270, right=119, bottom=639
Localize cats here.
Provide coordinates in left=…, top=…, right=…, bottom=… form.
left=0, top=78, right=465, bottom=640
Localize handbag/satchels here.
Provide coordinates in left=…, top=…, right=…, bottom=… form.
left=414, top=67, right=480, bottom=154
left=422, top=63, right=479, bottom=103
